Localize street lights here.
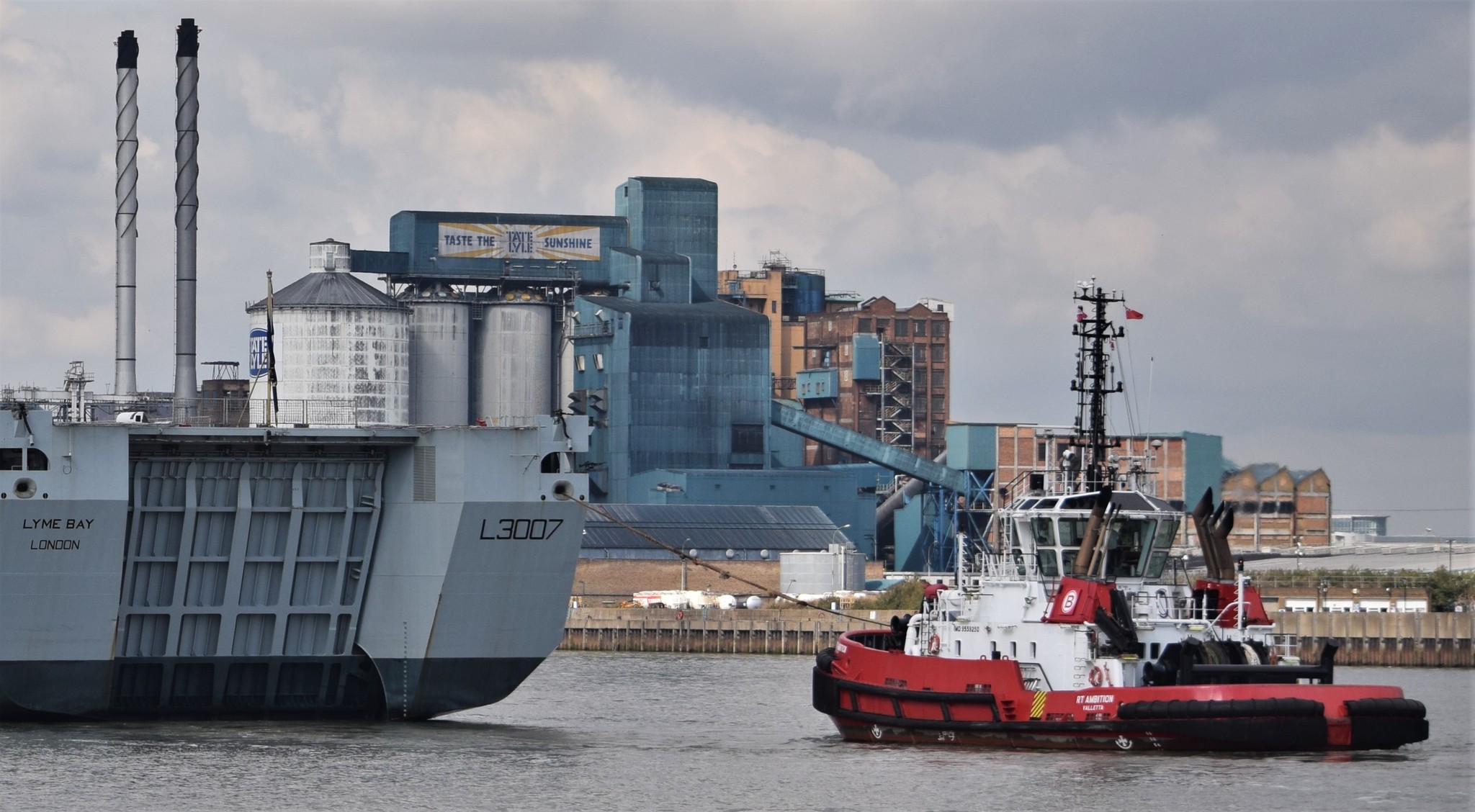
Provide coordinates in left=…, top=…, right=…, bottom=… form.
left=831, top=523, right=852, bottom=597
left=578, top=580, right=585, bottom=606
left=1425, top=528, right=1439, bottom=571
left=1292, top=536, right=1304, bottom=572
left=681, top=538, right=691, bottom=590
left=1444, top=539, right=1458, bottom=574
left=928, top=541, right=938, bottom=583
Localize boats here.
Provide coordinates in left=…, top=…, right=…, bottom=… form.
left=807, top=276, right=1430, bottom=755
left=0, top=16, right=594, bottom=726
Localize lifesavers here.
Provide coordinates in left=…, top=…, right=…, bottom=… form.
left=1089, top=666, right=1101, bottom=687
left=677, top=612, right=683, bottom=620
left=928, top=634, right=939, bottom=654
